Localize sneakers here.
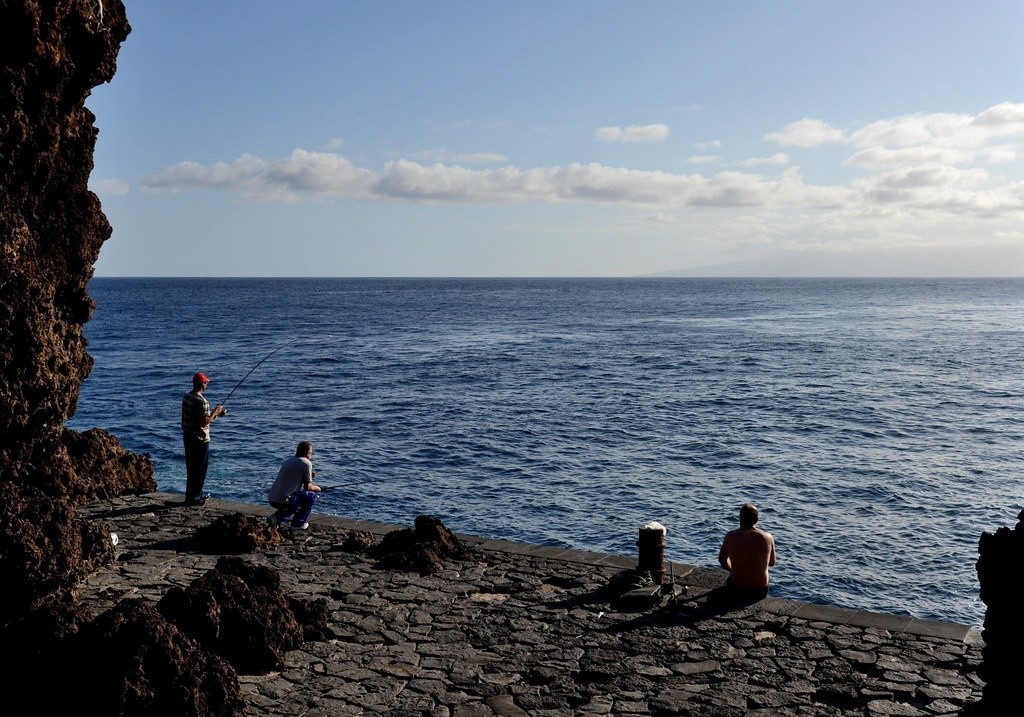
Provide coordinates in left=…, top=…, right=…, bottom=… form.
left=186, top=493, right=209, bottom=501
left=290, top=522, right=309, bottom=530
left=185, top=500, right=206, bottom=507
left=266, top=515, right=280, bottom=529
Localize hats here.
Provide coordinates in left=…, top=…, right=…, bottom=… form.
left=193, top=372, right=210, bottom=383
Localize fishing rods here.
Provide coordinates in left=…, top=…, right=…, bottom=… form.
left=213, top=339, right=298, bottom=420
left=315, top=477, right=397, bottom=492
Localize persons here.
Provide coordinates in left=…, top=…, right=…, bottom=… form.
left=719, top=504, right=776, bottom=598
left=267, top=441, right=321, bottom=530
left=182, top=373, right=222, bottom=506
